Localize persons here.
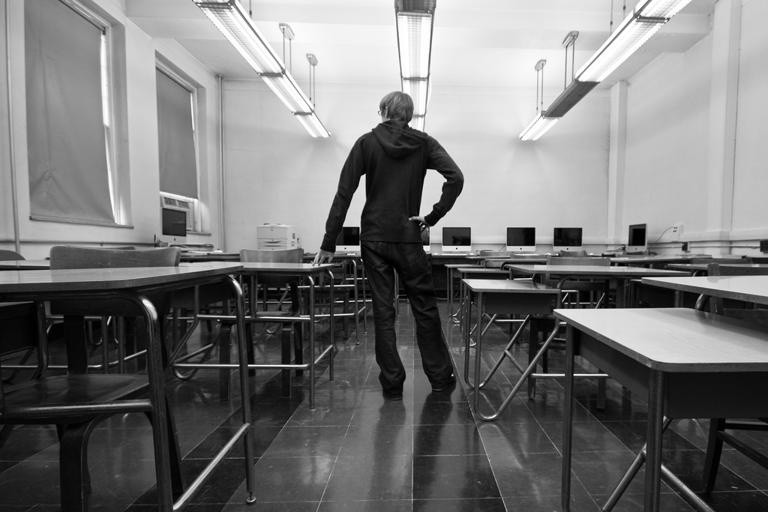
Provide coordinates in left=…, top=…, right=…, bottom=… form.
left=310, top=91, right=465, bottom=402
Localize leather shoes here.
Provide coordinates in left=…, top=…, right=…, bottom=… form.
left=432, top=374, right=455, bottom=392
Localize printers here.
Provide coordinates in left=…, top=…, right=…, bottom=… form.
left=257, top=223, right=302, bottom=251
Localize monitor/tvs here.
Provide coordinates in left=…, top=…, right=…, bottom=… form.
left=624, top=223, right=647, bottom=256
left=442, top=227, right=472, bottom=253
left=419, top=224, right=430, bottom=253
left=159, top=208, right=187, bottom=246
left=506, top=227, right=536, bottom=253
left=336, top=226, right=360, bottom=255
left=553, top=227, right=583, bottom=252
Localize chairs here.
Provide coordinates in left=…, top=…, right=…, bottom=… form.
left=420, top=235, right=768, bottom=512
left=0, top=236, right=396, bottom=512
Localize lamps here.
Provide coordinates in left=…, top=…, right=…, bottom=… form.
left=393, top=0, right=437, bottom=133
left=197, top=0, right=330, bottom=142
left=516, top=0, right=695, bottom=145
